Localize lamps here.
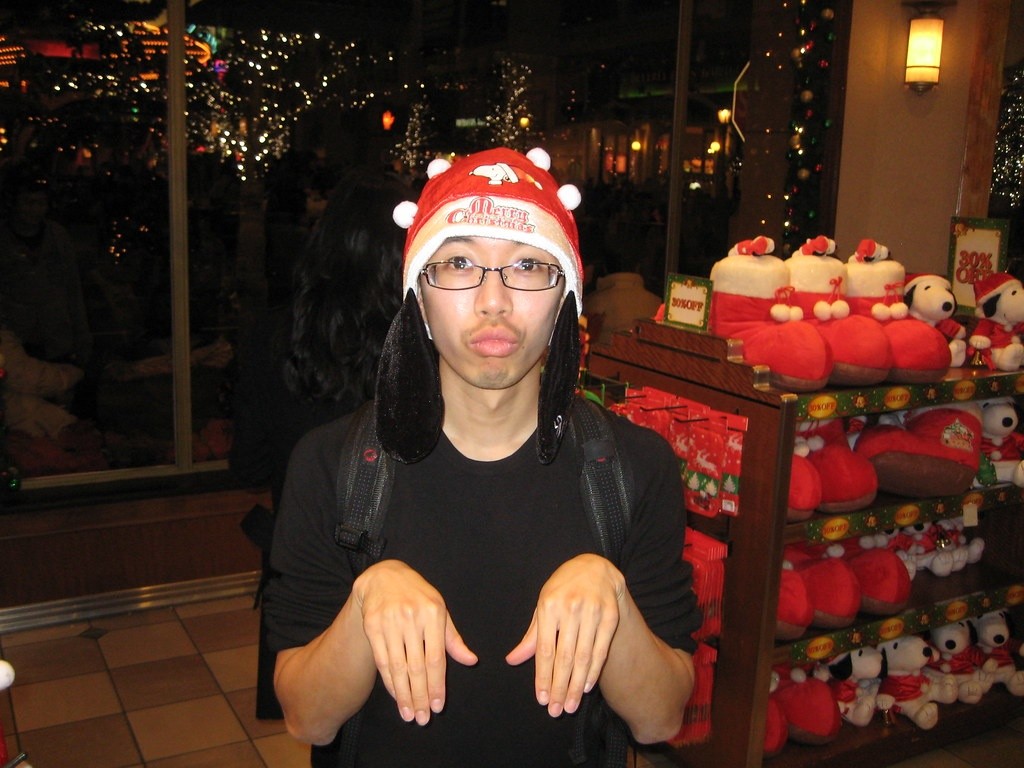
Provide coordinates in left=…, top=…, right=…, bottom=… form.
left=901, top=0, right=955, bottom=94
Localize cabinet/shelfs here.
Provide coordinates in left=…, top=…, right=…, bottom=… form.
left=589, top=318, right=1023, bottom=767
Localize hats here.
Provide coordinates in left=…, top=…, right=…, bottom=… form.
left=373, top=146, right=585, bottom=464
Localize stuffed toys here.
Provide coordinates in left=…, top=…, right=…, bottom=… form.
left=775, top=516, right=985, bottom=640
left=787, top=396, right=1024, bottom=521
left=711, top=236, right=1024, bottom=391
left=762, top=606, right=1024, bottom=758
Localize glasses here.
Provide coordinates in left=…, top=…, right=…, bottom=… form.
left=420, top=260, right=566, bottom=291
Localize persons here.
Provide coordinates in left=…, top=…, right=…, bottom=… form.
left=94, top=181, right=169, bottom=249
left=262, top=146, right=704, bottom=768
left=228, top=170, right=407, bottom=719
left=584, top=247, right=662, bottom=331
left=0, top=176, right=110, bottom=478
left=206, top=166, right=240, bottom=262
left=609, top=174, right=732, bottom=256
left=264, top=151, right=319, bottom=302
